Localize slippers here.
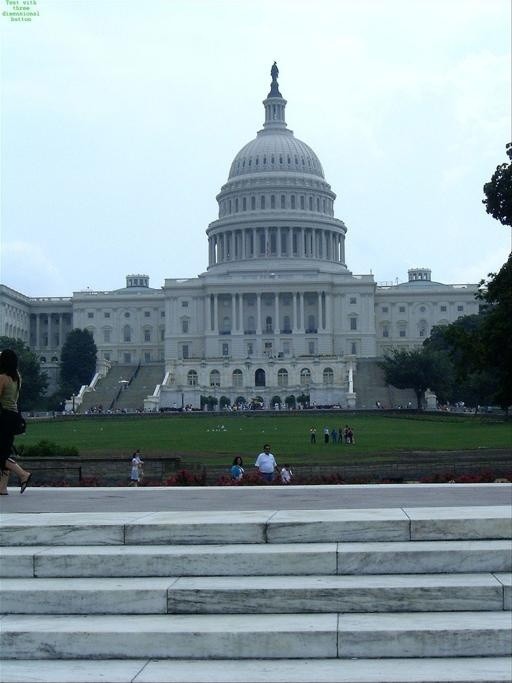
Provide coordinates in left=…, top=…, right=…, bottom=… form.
left=0, top=492, right=9, bottom=495
left=21, top=472, right=32, bottom=493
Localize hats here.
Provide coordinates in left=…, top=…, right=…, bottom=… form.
left=0, top=348, right=17, bottom=360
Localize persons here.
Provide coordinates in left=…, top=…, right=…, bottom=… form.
left=271, top=62, right=279, bottom=81
left=0, top=349, right=32, bottom=495
left=182, top=404, right=192, bottom=411
left=311, top=425, right=356, bottom=445
left=128, top=453, right=144, bottom=487
left=223, top=400, right=317, bottom=410
left=376, top=399, right=464, bottom=412
left=136, top=407, right=151, bottom=413
left=255, top=444, right=280, bottom=481
left=88, top=405, right=126, bottom=414
left=137, top=450, right=144, bottom=482
left=231, top=457, right=244, bottom=481
left=279, top=464, right=296, bottom=485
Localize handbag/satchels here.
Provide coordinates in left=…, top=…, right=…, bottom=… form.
left=14, top=413, right=25, bottom=434
